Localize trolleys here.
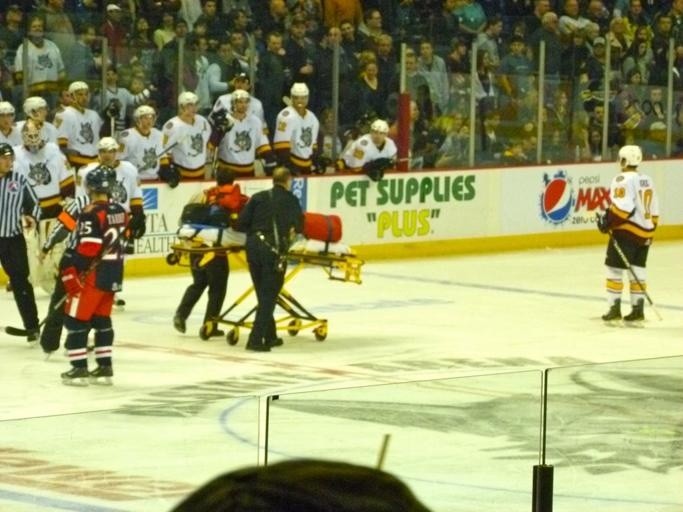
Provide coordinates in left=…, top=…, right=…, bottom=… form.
left=167, top=238, right=362, bottom=348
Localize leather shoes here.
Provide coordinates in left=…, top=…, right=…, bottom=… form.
left=246, top=343, right=271, bottom=352
left=173, top=316, right=186, bottom=333
left=201, top=330, right=224, bottom=336
left=268, top=337, right=283, bottom=347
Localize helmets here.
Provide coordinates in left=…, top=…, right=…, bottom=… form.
left=86, top=164, right=117, bottom=192
left=0, top=142, right=15, bottom=158
left=371, top=118, right=390, bottom=135
left=21, top=118, right=40, bottom=146
left=617, top=143, right=644, bottom=170
left=24, top=96, right=48, bottom=115
left=67, top=80, right=90, bottom=95
left=1, top=100, right=18, bottom=117
left=96, top=136, right=120, bottom=153
left=134, top=83, right=309, bottom=131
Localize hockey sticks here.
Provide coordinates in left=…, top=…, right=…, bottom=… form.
left=583, top=209, right=666, bottom=322
left=4, top=213, right=146, bottom=336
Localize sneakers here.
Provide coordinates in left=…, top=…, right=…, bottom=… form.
left=60, top=365, right=90, bottom=378
left=601, top=300, right=621, bottom=319
left=89, top=364, right=113, bottom=377
left=623, top=299, right=644, bottom=320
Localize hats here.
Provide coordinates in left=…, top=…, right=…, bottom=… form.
left=233, top=72, right=250, bottom=83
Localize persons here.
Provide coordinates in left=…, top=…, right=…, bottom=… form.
left=74, top=136, right=146, bottom=312
left=59, top=164, right=131, bottom=387
left=1, top=1, right=682, bottom=200
left=233, top=166, right=305, bottom=353
left=173, top=163, right=237, bottom=341
left=37, top=197, right=80, bottom=361
left=597, top=145, right=659, bottom=328
left=0, top=143, right=41, bottom=348
left=167, top=458, right=432, bottom=511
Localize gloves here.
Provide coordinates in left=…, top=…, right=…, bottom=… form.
left=211, top=109, right=231, bottom=144
left=59, top=266, right=83, bottom=296
left=157, top=161, right=180, bottom=190
left=262, top=152, right=280, bottom=177
left=360, top=156, right=393, bottom=181
left=129, top=209, right=145, bottom=238
left=596, top=215, right=612, bottom=233
